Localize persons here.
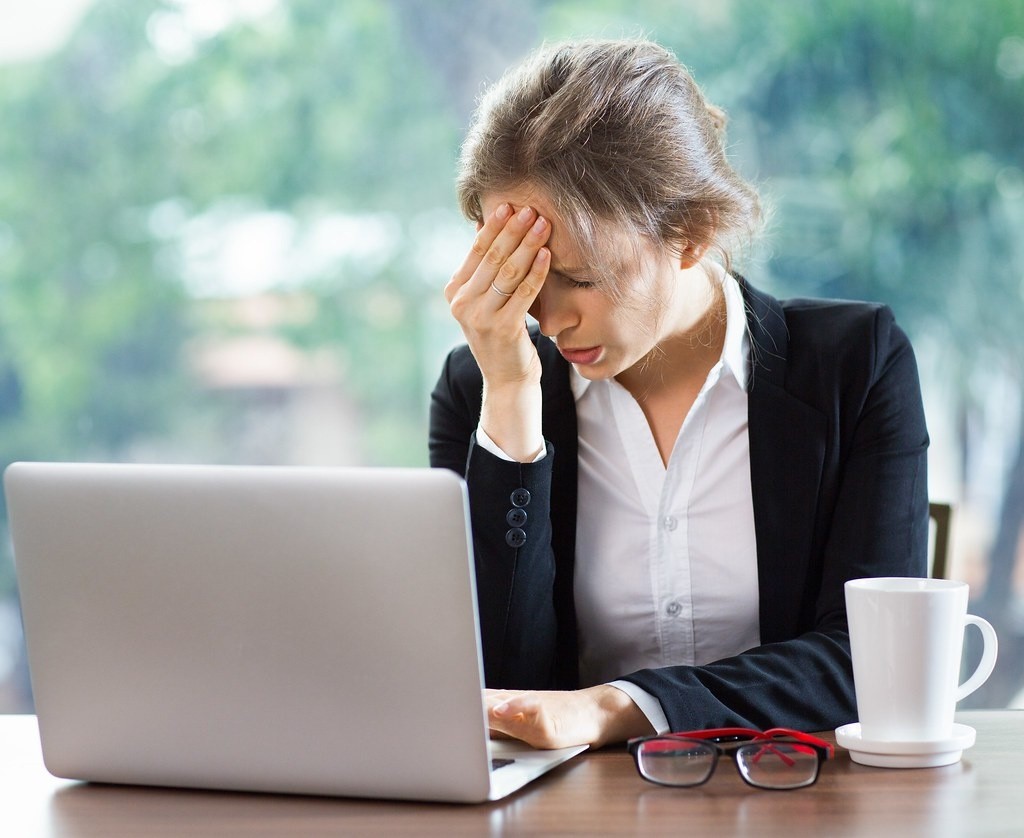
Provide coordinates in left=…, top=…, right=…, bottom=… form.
left=426, top=29, right=931, bottom=752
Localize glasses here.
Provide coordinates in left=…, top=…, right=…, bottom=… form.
left=627, top=728, right=835, bottom=790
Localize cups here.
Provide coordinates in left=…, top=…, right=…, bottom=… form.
left=844, top=576, right=999, bottom=744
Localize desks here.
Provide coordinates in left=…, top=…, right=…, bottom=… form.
left=0, top=712, right=1024, bottom=838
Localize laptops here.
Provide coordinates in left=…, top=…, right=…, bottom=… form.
left=5, top=460, right=591, bottom=804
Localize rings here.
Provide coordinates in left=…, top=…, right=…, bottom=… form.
left=490, top=281, right=513, bottom=298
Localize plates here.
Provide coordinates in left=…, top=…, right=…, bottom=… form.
left=834, top=721, right=976, bottom=769
